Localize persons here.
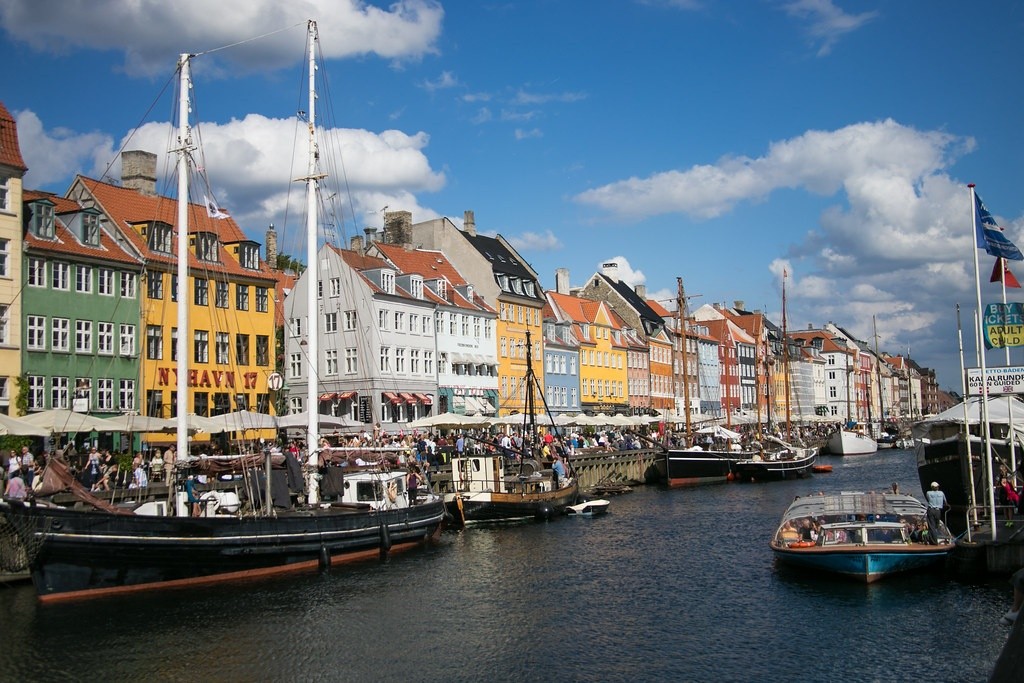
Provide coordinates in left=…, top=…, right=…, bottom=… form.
left=778, top=481, right=947, bottom=547
left=0, top=437, right=304, bottom=517
left=320, top=425, right=835, bottom=506
left=997, top=464, right=1020, bottom=527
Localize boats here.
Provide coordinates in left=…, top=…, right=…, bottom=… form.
left=564, top=499, right=610, bottom=519
left=910, top=394, right=1024, bottom=538
left=769, top=483, right=956, bottom=584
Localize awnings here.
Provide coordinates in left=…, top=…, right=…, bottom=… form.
left=383, top=392, right=431, bottom=405
left=340, top=392, right=357, bottom=400
left=319, top=393, right=338, bottom=401
left=453, top=396, right=495, bottom=415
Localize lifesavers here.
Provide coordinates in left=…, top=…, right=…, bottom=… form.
left=788, top=542, right=815, bottom=548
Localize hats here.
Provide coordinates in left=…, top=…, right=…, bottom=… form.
left=931, top=482, right=940, bottom=488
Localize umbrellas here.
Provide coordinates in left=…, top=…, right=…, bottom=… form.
left=0, top=408, right=367, bottom=449
left=407, top=411, right=847, bottom=431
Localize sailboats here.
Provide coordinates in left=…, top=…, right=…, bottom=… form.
left=0, top=19, right=450, bottom=608
left=826, top=315, right=915, bottom=455
left=652, top=269, right=819, bottom=485
left=442, top=319, right=579, bottom=528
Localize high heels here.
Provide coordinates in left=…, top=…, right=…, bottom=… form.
left=1006, top=521, right=1013, bottom=527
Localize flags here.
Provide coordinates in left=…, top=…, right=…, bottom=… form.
left=973, top=190, right=1024, bottom=352
left=204, top=195, right=230, bottom=219
